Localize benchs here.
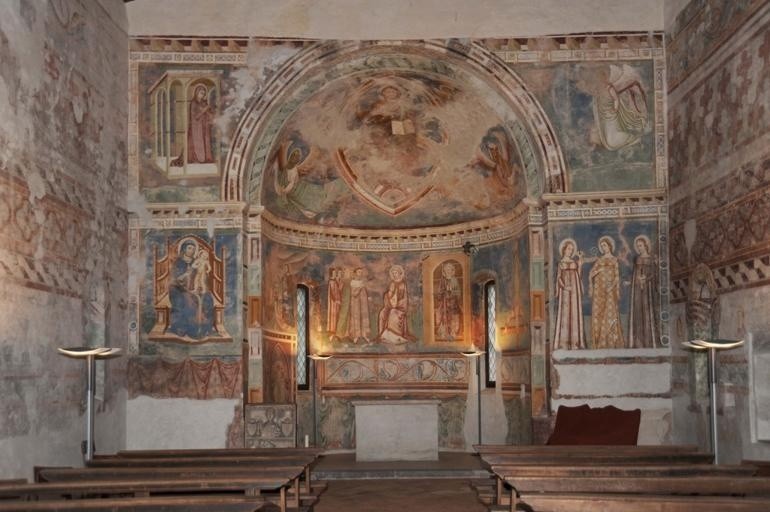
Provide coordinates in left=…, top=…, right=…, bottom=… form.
left=472, top=442, right=770, bottom=512
left=1, top=447, right=331, bottom=510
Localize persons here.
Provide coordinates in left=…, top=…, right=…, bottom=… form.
left=168, top=84, right=213, bottom=167
left=433, top=262, right=463, bottom=339
left=553, top=237, right=654, bottom=351
left=351, top=84, right=440, bottom=177
left=168, top=239, right=216, bottom=338
left=273, top=261, right=408, bottom=342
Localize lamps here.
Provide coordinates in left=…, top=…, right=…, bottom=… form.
left=57, top=343, right=120, bottom=460
left=681, top=338, right=745, bottom=462
left=461, top=350, right=488, bottom=447
left=304, top=355, right=331, bottom=457
